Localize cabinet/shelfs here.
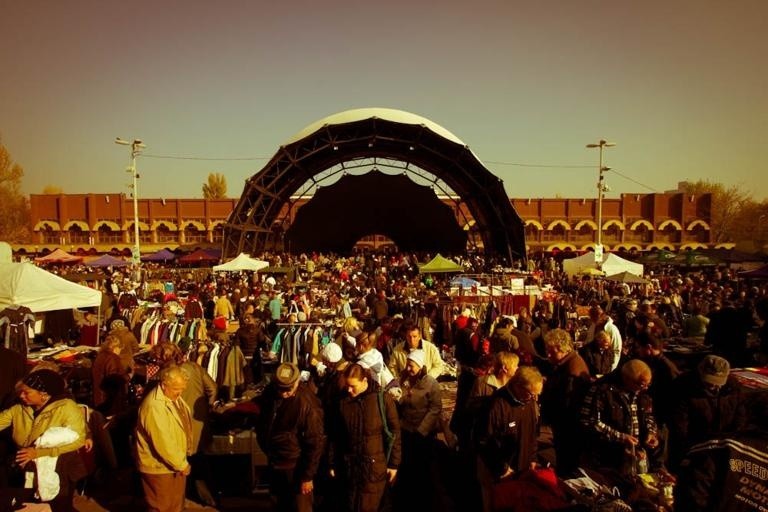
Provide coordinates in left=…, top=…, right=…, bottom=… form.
left=28, top=347, right=161, bottom=404
left=661, top=335, right=759, bottom=356
left=200, top=430, right=255, bottom=494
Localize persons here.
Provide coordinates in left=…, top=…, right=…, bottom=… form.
left=479, top=441, right=567, bottom=512
left=581, top=359, right=658, bottom=485
left=147, top=342, right=220, bottom=511
left=470, top=351, right=519, bottom=398
left=321, top=341, right=355, bottom=415
left=329, top=363, right=402, bottom=512
left=234, top=314, right=272, bottom=385
left=356, top=332, right=402, bottom=402
left=93, top=336, right=132, bottom=420
left=256, top=363, right=325, bottom=512
left=0, top=369, right=92, bottom=512
left=445, top=252, right=768, bottom=376
left=386, top=251, right=443, bottom=322
left=473, top=365, right=546, bottom=512
left=11, top=245, right=387, bottom=335
left=675, top=354, right=748, bottom=444
left=666, top=431, right=768, bottom=512
left=133, top=367, right=194, bottom=512
left=107, top=320, right=139, bottom=379
left=390, top=323, right=443, bottom=381
left=93, top=333, right=135, bottom=467
left=399, top=350, right=442, bottom=450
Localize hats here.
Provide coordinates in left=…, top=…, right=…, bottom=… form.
left=22, top=369, right=65, bottom=397
left=212, top=316, right=226, bottom=331
left=406, top=349, right=426, bottom=369
left=698, top=355, right=730, bottom=386
left=378, top=289, right=387, bottom=298
left=319, top=343, right=343, bottom=363
left=275, top=361, right=301, bottom=387
left=108, top=320, right=128, bottom=334
left=296, top=311, right=307, bottom=321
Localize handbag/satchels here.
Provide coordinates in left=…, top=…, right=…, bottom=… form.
left=376, top=386, right=399, bottom=468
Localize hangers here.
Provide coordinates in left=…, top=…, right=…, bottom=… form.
left=191, top=340, right=240, bottom=398
left=274, top=322, right=333, bottom=343
left=144, top=319, right=206, bottom=323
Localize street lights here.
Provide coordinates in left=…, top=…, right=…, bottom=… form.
left=586, top=138, right=616, bottom=248
left=116, top=139, right=145, bottom=246
